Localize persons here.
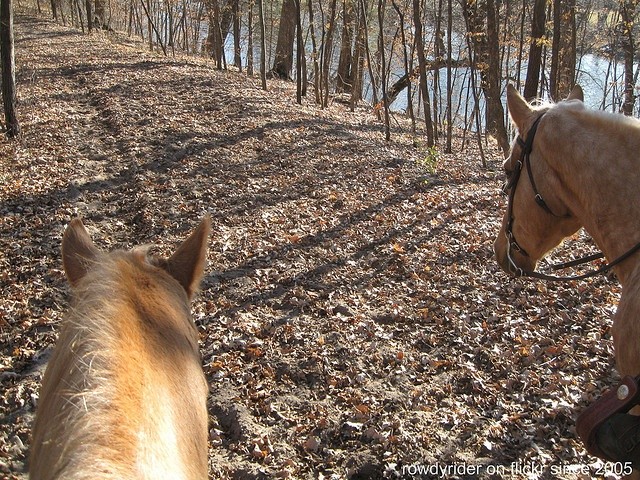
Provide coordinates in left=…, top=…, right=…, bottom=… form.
left=590, top=400, right=620, bottom=463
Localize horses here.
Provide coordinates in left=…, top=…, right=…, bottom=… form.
left=493, top=83, right=640, bottom=480
left=29, top=213, right=210, bottom=480
